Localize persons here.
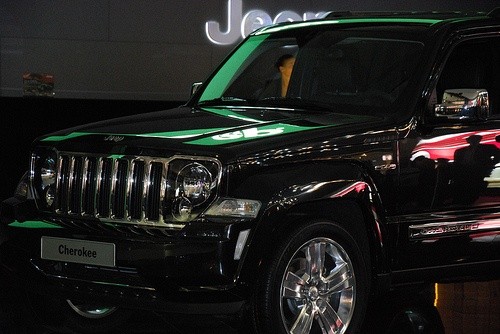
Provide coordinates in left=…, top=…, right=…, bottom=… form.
left=260, top=54, right=296, bottom=100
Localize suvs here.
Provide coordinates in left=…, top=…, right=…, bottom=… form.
left=0, top=11, right=500, bottom=334
